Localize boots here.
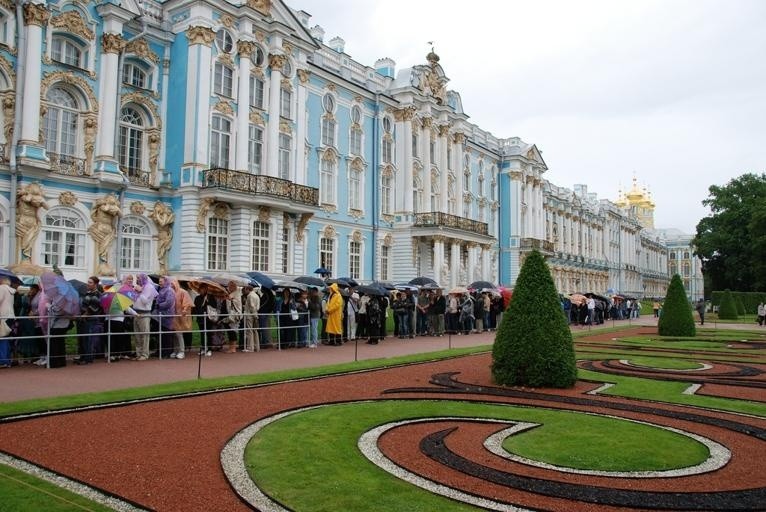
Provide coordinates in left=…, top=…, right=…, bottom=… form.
left=224, top=341, right=236, bottom=354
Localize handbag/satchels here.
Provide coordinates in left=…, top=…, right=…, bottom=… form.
left=207, top=305, right=218, bottom=323
left=228, top=309, right=243, bottom=324
left=0, top=318, right=12, bottom=337
left=291, top=309, right=298, bottom=320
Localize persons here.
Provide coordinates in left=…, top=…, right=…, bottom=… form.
left=1, top=269, right=505, bottom=368
left=758, top=302, right=765, bottom=325
left=764, top=304, right=766, bottom=325
left=654, top=299, right=665, bottom=317
left=563, top=294, right=641, bottom=325
left=696, top=298, right=720, bottom=325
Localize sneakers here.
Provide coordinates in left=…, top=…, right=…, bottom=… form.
left=170, top=351, right=185, bottom=359
left=33, top=356, right=47, bottom=365
